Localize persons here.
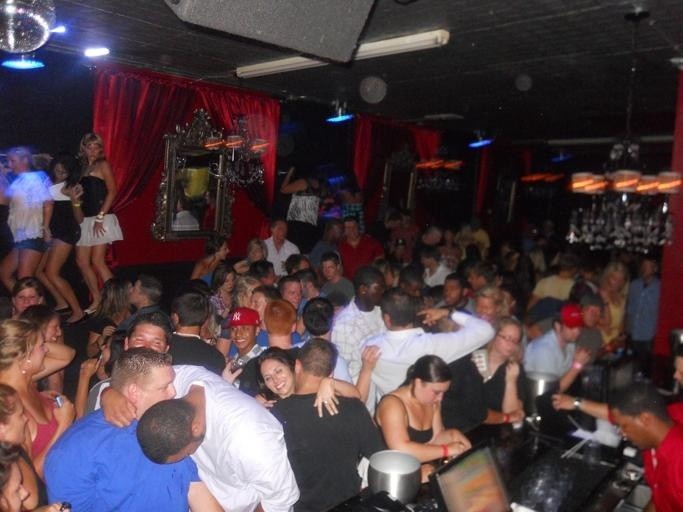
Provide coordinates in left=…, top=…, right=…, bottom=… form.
left=203, top=190, right=216, bottom=231
left=1, top=133, right=124, bottom=326
left=172, top=196, right=200, bottom=230
left=1, top=205, right=682, bottom=512
left=281, top=151, right=366, bottom=237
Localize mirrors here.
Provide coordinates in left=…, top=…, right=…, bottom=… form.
left=151, top=107, right=236, bottom=244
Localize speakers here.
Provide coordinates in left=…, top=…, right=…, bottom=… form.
left=163, top=0, right=378, bottom=68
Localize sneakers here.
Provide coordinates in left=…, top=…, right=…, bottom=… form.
left=49, top=306, right=97, bottom=328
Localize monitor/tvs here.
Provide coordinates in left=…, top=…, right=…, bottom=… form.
left=428, top=436, right=511, bottom=512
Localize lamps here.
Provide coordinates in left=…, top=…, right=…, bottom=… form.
left=205, top=63, right=268, bottom=157
left=236, top=30, right=449, bottom=80
left=567, top=9, right=683, bottom=196
left=0, top=0, right=56, bottom=54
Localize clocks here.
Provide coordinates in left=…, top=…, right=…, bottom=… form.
left=359, top=76, right=389, bottom=105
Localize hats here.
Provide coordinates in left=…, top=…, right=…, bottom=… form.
left=553, top=302, right=588, bottom=329
left=223, top=307, right=261, bottom=329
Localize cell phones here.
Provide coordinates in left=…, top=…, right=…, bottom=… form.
left=55, top=396, right=63, bottom=407
